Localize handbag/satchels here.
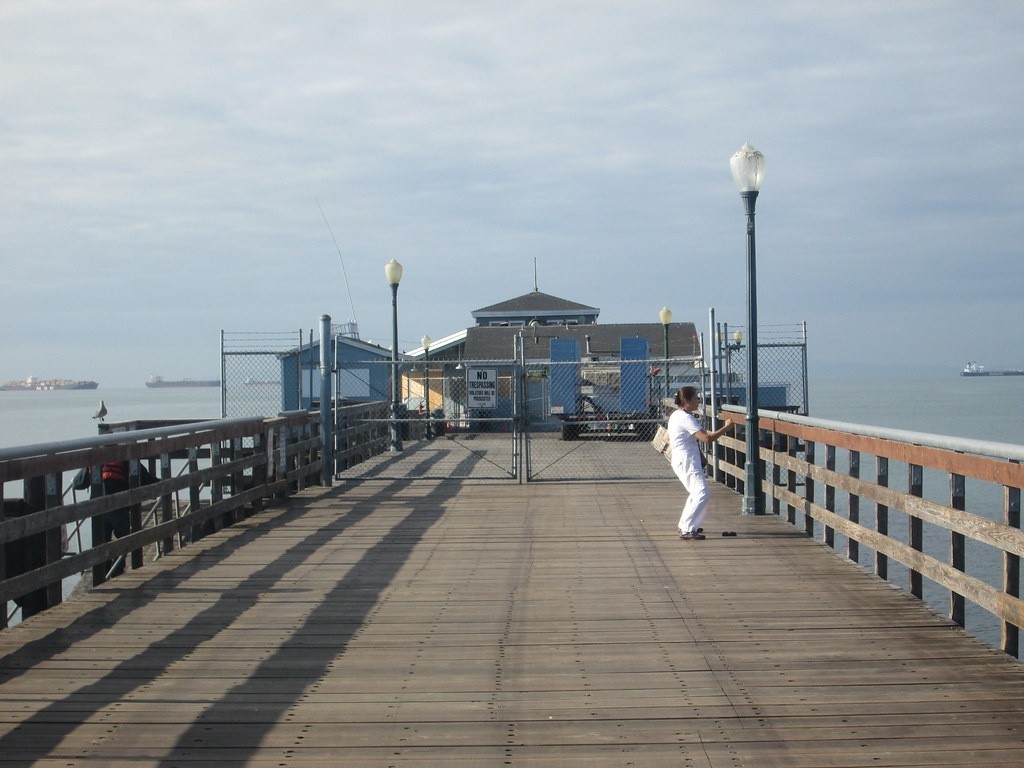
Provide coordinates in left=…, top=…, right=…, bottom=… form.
left=700, top=449, right=708, bottom=469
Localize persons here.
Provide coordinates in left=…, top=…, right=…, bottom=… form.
left=72, top=431, right=161, bottom=588
left=663, top=386, right=733, bottom=540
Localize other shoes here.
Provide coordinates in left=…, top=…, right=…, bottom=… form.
left=679, top=528, right=705, bottom=540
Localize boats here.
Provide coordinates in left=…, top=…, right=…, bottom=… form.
left=0, top=380, right=99, bottom=389
left=145, top=376, right=220, bottom=388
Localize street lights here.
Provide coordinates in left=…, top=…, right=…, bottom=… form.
left=421, top=334, right=432, bottom=441
left=659, top=305, right=673, bottom=427
left=717, top=330, right=742, bottom=402
left=384, top=258, right=403, bottom=454
left=728, top=140, right=769, bottom=517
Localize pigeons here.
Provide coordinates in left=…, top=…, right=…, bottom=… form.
left=92, top=401, right=108, bottom=423
